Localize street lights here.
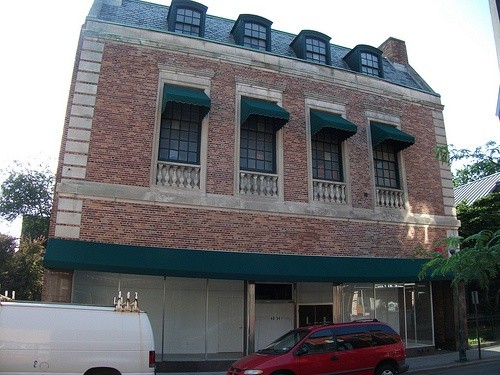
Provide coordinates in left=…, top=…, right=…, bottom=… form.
left=450, top=248, right=468, bottom=361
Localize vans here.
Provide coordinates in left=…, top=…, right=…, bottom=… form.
left=0, top=301, right=157, bottom=375
left=227, top=318, right=405, bottom=375
